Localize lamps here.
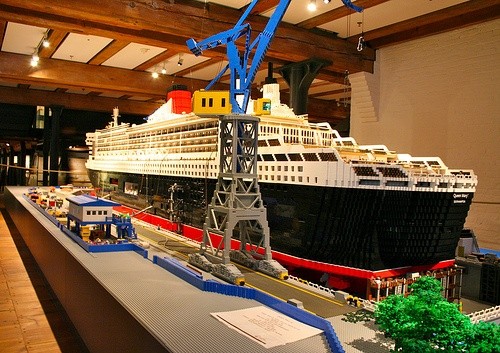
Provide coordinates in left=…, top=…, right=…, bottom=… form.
left=177, top=51, right=184, bottom=66
left=66, top=143, right=73, bottom=149
left=152, top=64, right=158, bottom=79
left=42, top=32, right=49, bottom=48
left=324, top=0, right=331, bottom=5
left=160, top=59, right=169, bottom=74
left=30, top=48, right=39, bottom=66
left=307, top=0, right=317, bottom=10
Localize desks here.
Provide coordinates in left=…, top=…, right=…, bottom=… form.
left=454, top=257, right=500, bottom=308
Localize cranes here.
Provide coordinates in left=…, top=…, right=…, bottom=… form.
left=186, top=0, right=365, bottom=286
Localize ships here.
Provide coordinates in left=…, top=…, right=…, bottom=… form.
left=85, top=60, right=479, bottom=300
